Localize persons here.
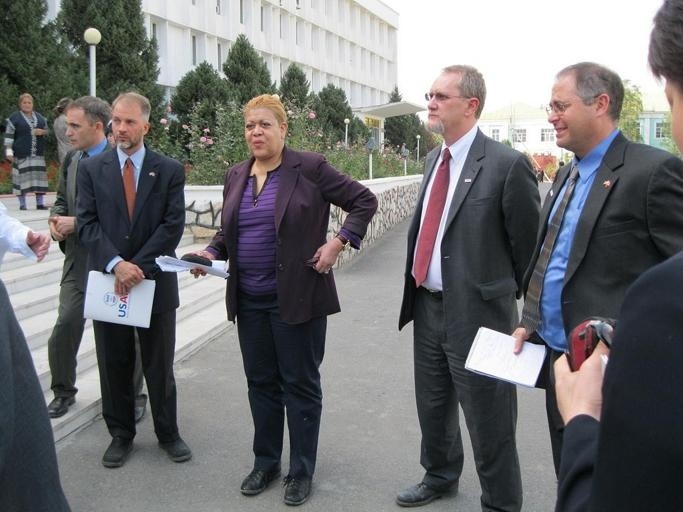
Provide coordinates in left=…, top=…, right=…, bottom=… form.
left=559, top=161, right=564, bottom=167
left=395, top=65, right=542, bottom=512
left=510, top=62, right=683, bottom=479
left=551, top=0, right=683, bottom=512
left=4, top=93, right=49, bottom=210
left=534, top=165, right=544, bottom=183
left=46, top=94, right=147, bottom=424
left=75, top=92, right=192, bottom=468
left=106, top=126, right=115, bottom=145
left=0, top=201, right=71, bottom=512
left=190, top=94, right=378, bottom=507
left=51, top=97, right=74, bottom=166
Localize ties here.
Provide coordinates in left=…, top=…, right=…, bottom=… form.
left=123, top=158, right=136, bottom=217
left=522, top=166, right=579, bottom=335
left=414, top=148, right=450, bottom=288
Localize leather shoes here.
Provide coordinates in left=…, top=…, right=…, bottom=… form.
left=283, top=471, right=312, bottom=506
left=20, top=206, right=26, bottom=210
left=102, top=438, right=134, bottom=468
left=48, top=397, right=75, bottom=418
left=37, top=205, right=48, bottom=209
left=240, top=468, right=282, bottom=495
left=158, top=437, right=192, bottom=462
left=395, top=482, right=459, bottom=507
left=135, top=406, right=144, bottom=422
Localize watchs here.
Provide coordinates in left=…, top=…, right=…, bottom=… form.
left=336, top=234, right=350, bottom=249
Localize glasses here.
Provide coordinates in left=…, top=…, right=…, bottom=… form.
left=546, top=103, right=566, bottom=114
left=425, top=92, right=470, bottom=100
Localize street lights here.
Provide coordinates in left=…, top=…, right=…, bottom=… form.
left=84, top=25, right=103, bottom=98
left=416, top=134, right=421, bottom=160
left=343, top=117, right=350, bottom=144
